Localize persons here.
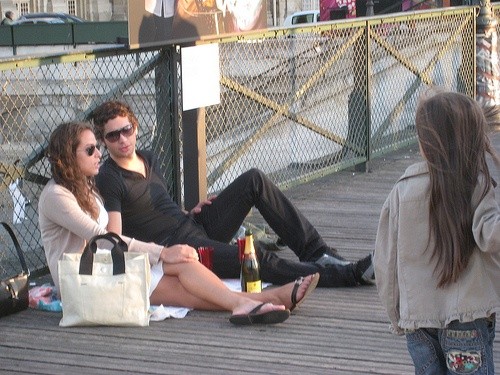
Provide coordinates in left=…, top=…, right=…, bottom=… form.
left=373, top=92, right=500, bottom=375
left=3, top=11, right=14, bottom=24
left=93, top=101, right=374, bottom=286
left=38, top=122, right=320, bottom=324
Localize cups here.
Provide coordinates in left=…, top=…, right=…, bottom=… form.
left=237, top=237, right=248, bottom=263
left=197, top=246, right=215, bottom=272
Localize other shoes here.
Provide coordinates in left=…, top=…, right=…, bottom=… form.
left=356, top=250, right=375, bottom=282
left=310, top=246, right=354, bottom=266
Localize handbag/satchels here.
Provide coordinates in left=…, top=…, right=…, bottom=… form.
left=0, top=222, right=30, bottom=317
left=57, top=231, right=151, bottom=328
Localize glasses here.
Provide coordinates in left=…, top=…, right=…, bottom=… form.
left=102, top=124, right=134, bottom=143
left=76, top=142, right=101, bottom=156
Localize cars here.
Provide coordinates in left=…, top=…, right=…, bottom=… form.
left=12, top=13, right=85, bottom=25
left=283, top=10, right=322, bottom=39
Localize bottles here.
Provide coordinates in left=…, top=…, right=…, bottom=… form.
left=239, top=229, right=262, bottom=294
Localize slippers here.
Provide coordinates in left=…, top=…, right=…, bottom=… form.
left=229, top=302, right=291, bottom=324
left=289, top=272, right=319, bottom=314
